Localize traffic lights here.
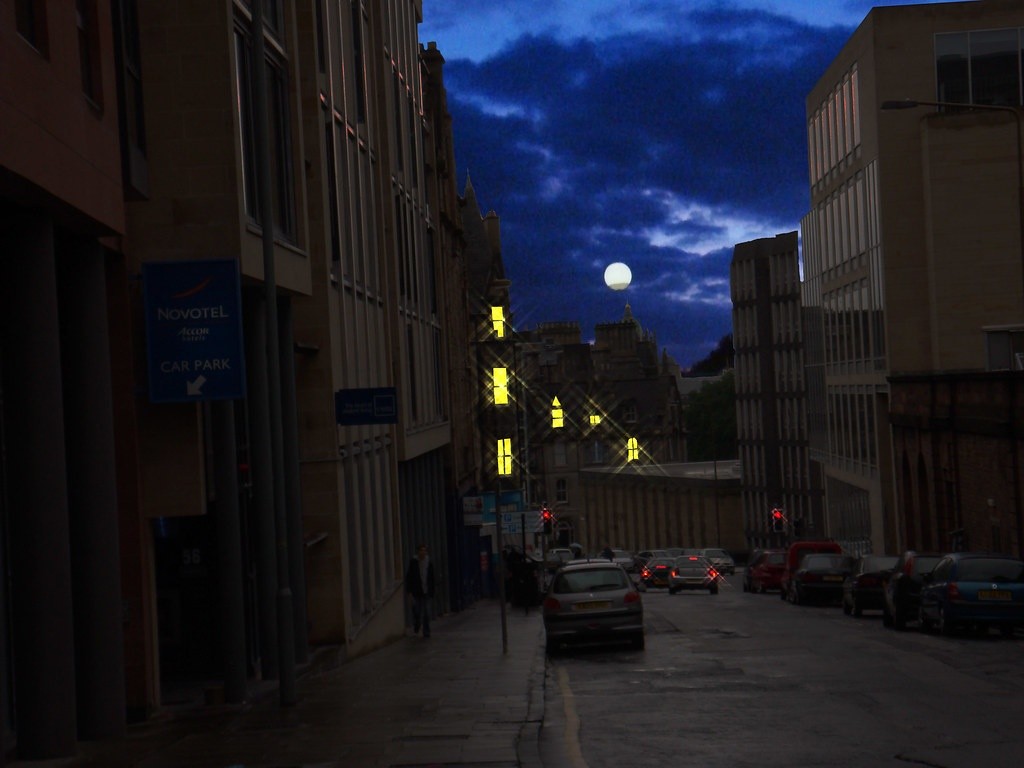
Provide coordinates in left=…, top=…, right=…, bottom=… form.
left=543, top=508, right=553, bottom=536
left=773, top=509, right=784, bottom=533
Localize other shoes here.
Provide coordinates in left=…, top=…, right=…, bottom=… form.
left=414, top=624, right=420, bottom=633
left=424, top=631, right=430, bottom=639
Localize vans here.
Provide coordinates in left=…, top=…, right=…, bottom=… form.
left=779, top=541, right=841, bottom=601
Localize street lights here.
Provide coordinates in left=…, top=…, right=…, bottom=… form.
left=879, top=98, right=1024, bottom=263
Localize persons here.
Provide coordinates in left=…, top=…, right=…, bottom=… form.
left=406, top=544, right=436, bottom=637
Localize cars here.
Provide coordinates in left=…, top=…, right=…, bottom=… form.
left=543, top=563, right=645, bottom=655
left=744, top=549, right=763, bottom=593
left=520, top=553, right=544, bottom=571
left=634, top=548, right=689, bottom=588
left=549, top=549, right=576, bottom=562
left=666, top=554, right=719, bottom=594
left=916, top=553, right=1023, bottom=637
left=596, top=549, right=634, bottom=573
left=697, top=548, right=735, bottom=577
left=786, top=552, right=858, bottom=606
left=878, top=550, right=951, bottom=633
left=842, top=552, right=905, bottom=620
left=749, top=550, right=787, bottom=595
